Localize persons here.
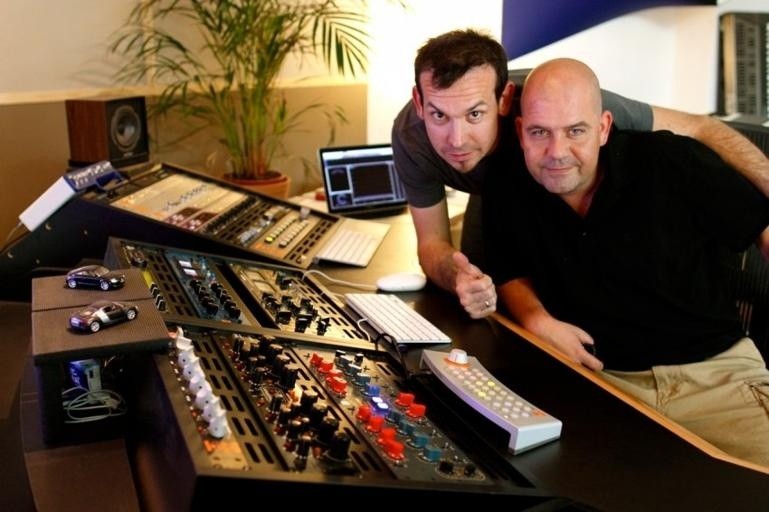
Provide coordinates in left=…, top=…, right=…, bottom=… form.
left=486, top=59, right=769, bottom=472
left=389, top=27, right=769, bottom=321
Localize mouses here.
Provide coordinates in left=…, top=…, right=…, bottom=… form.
left=377, top=273, right=427, bottom=292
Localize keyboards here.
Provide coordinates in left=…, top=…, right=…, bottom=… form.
left=312, top=217, right=391, bottom=268
left=345, top=293, right=453, bottom=350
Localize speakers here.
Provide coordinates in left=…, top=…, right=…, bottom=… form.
left=65, top=95, right=149, bottom=169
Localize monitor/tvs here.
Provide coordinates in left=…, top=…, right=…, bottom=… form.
left=318, top=144, right=408, bottom=215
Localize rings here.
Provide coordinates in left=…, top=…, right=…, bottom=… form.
left=485, top=301, right=489, bottom=307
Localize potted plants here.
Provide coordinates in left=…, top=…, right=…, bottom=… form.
left=101, top=1, right=410, bottom=205
